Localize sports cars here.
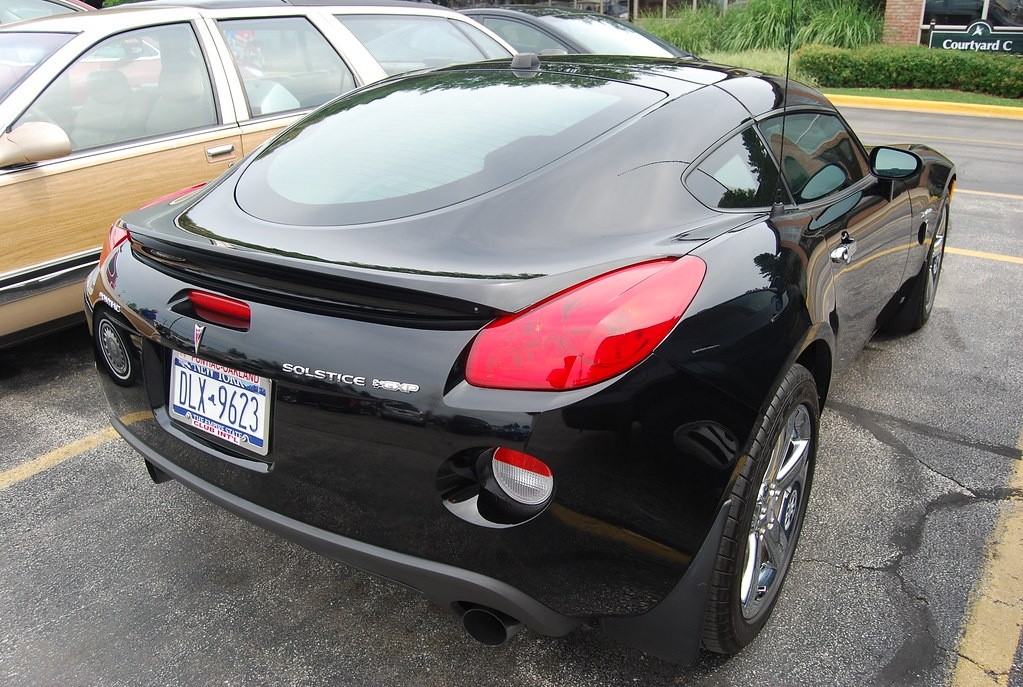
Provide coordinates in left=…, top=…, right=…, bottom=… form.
left=81, top=49, right=957, bottom=663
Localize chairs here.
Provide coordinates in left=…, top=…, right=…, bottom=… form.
left=147, top=47, right=205, bottom=134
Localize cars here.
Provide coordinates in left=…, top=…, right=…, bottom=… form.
left=0, top=0, right=269, bottom=94
left=333, top=8, right=691, bottom=63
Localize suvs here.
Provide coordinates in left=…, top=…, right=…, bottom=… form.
left=0, top=5, right=519, bottom=348
